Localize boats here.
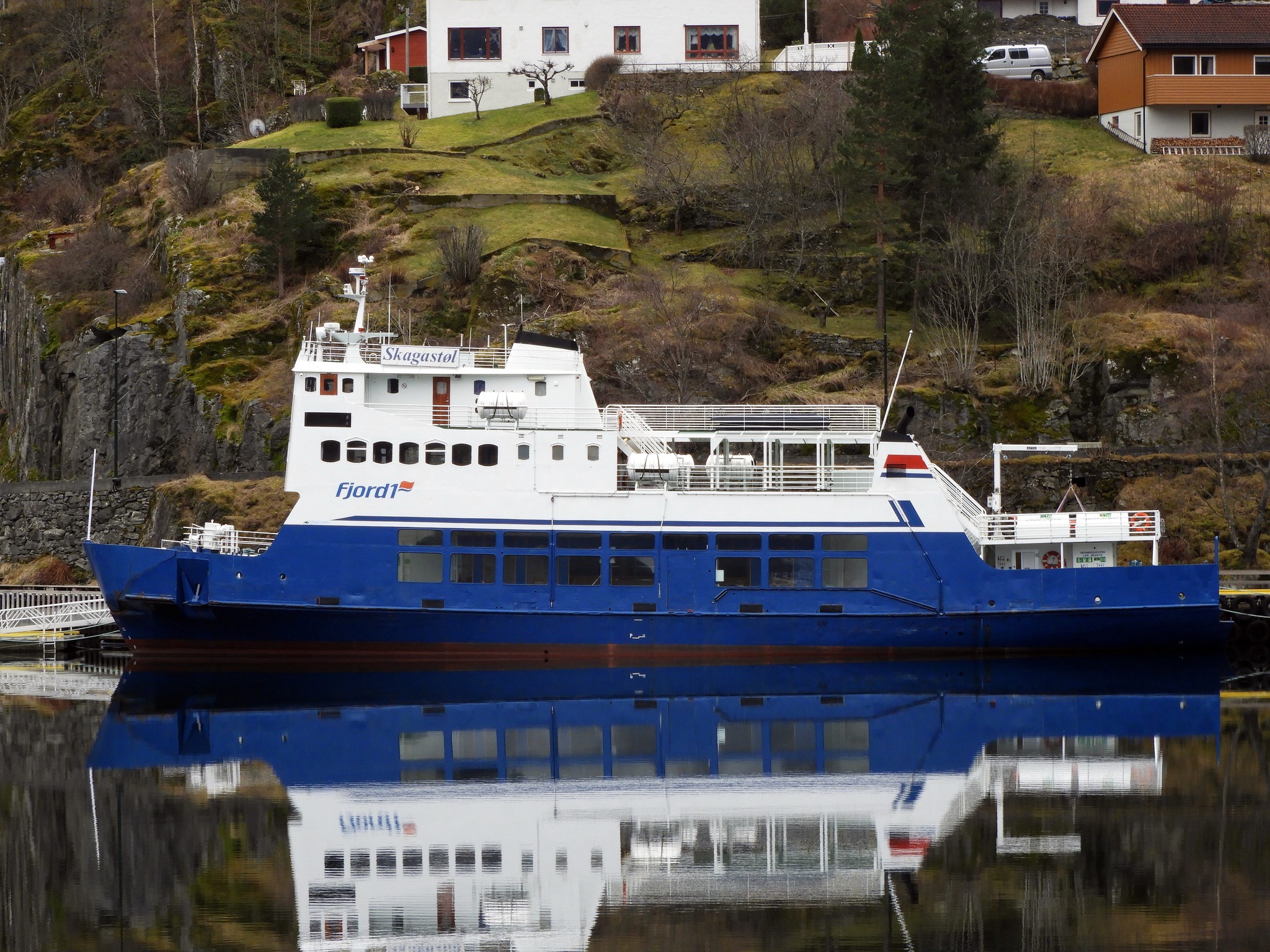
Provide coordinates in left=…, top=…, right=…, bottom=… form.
left=85, top=632, right=1269, bottom=952
left=79, top=254, right=1269, bottom=661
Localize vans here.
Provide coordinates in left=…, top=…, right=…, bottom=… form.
left=972, top=44, right=1054, bottom=84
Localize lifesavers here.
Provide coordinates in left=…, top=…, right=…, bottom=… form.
left=1248, top=620, right=1270, bottom=643
left=1130, top=513, right=1151, bottom=531
left=1262, top=596, right=1270, bottom=618
left=1043, top=551, right=1061, bottom=569
left=618, top=409, right=623, bottom=431
left=1218, top=597, right=1227, bottom=616
left=1230, top=594, right=1258, bottom=622
